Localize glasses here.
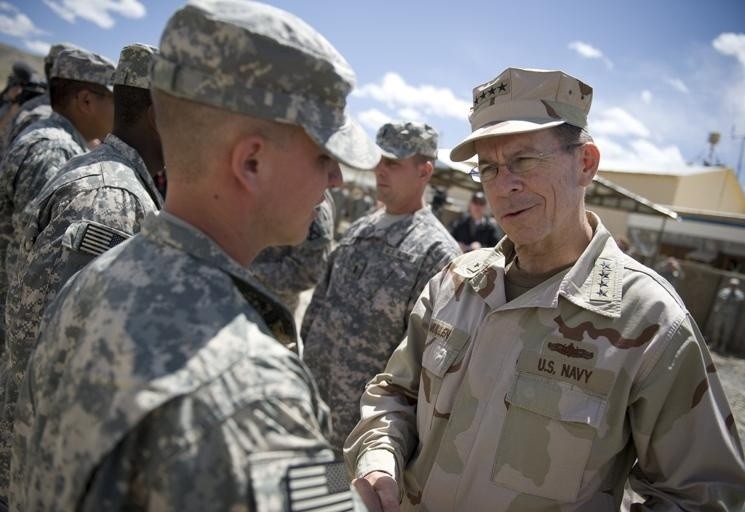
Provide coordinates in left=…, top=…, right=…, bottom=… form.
left=468, top=144, right=584, bottom=183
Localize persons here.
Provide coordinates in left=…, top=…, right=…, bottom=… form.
left=706, top=277, right=745, bottom=356
left=651, top=255, right=685, bottom=293
left=0, top=44, right=162, bottom=511
left=6, top=0, right=358, bottom=511
left=1, top=43, right=503, bottom=258
left=343, top=67, right=745, bottom=510
left=300, top=124, right=461, bottom=463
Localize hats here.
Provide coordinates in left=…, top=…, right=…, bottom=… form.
left=46, top=43, right=159, bottom=90
left=450, top=68, right=594, bottom=162
left=149, top=0, right=382, bottom=170
left=375, top=121, right=439, bottom=160
